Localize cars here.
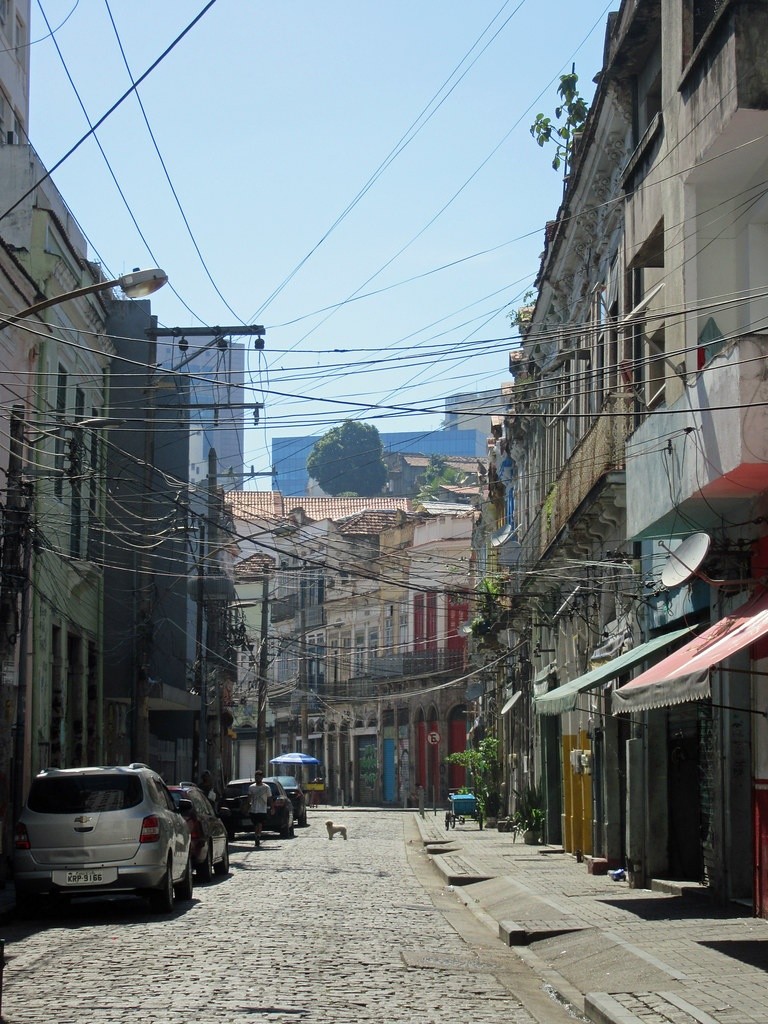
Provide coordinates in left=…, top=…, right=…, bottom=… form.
left=275, top=776, right=307, bottom=827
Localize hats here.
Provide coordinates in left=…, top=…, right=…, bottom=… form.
left=201, top=770, right=210, bottom=778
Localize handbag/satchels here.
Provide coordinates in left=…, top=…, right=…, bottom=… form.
left=208, top=785, right=217, bottom=801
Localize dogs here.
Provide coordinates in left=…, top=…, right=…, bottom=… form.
left=325, top=820, right=348, bottom=840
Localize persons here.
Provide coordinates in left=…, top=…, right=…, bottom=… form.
left=246, top=770, right=276, bottom=847
left=198, top=769, right=219, bottom=818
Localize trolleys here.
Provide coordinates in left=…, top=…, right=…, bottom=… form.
left=445, top=792, right=483, bottom=831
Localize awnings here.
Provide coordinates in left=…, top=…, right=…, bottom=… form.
left=535, top=617, right=711, bottom=716
left=611, top=591, right=768, bottom=717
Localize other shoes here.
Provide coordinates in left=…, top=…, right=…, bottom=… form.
left=255, top=840, right=260, bottom=847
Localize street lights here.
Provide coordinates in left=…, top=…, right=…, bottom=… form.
left=255, top=622, right=346, bottom=778
left=128, top=524, right=298, bottom=758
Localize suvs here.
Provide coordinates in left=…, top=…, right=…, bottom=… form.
left=165, top=782, right=230, bottom=881
left=219, top=777, right=293, bottom=837
left=12, top=763, right=194, bottom=911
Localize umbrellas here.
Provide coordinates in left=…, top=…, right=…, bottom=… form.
left=271, top=752, right=320, bottom=789
